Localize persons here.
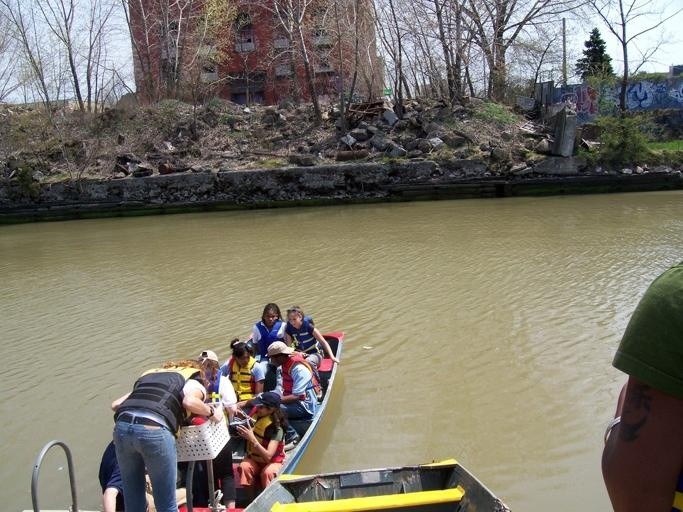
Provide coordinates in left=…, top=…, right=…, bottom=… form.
left=285, top=305, right=340, bottom=384
left=265, top=341, right=319, bottom=450
left=251, top=303, right=287, bottom=390
left=199, top=350, right=237, bottom=509
left=600, top=260, right=683, bottom=512
left=110, top=359, right=223, bottom=512
left=235, top=392, right=285, bottom=503
left=98, top=439, right=187, bottom=512
left=220, top=338, right=265, bottom=403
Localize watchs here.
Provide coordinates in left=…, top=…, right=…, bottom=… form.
left=207, top=406, right=214, bottom=417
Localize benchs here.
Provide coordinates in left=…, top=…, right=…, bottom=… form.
left=270, top=485, right=466, bottom=512
left=178, top=358, right=334, bottom=512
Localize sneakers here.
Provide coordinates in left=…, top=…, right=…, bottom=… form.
left=284, top=434, right=301, bottom=451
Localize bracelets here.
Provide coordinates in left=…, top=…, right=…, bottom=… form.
left=254, top=442, right=259, bottom=447
left=603, top=416, right=621, bottom=444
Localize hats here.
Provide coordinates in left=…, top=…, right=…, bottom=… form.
left=199, top=350, right=218, bottom=362
left=250, top=392, right=281, bottom=406
left=267, top=341, right=295, bottom=356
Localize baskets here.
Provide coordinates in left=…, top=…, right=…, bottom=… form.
left=176, top=402, right=230, bottom=462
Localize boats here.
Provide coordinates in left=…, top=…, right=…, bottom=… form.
left=93, top=331, right=345, bottom=511
left=245, top=441, right=509, bottom=512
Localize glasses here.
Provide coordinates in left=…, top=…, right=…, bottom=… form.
left=286, top=310, right=296, bottom=313
